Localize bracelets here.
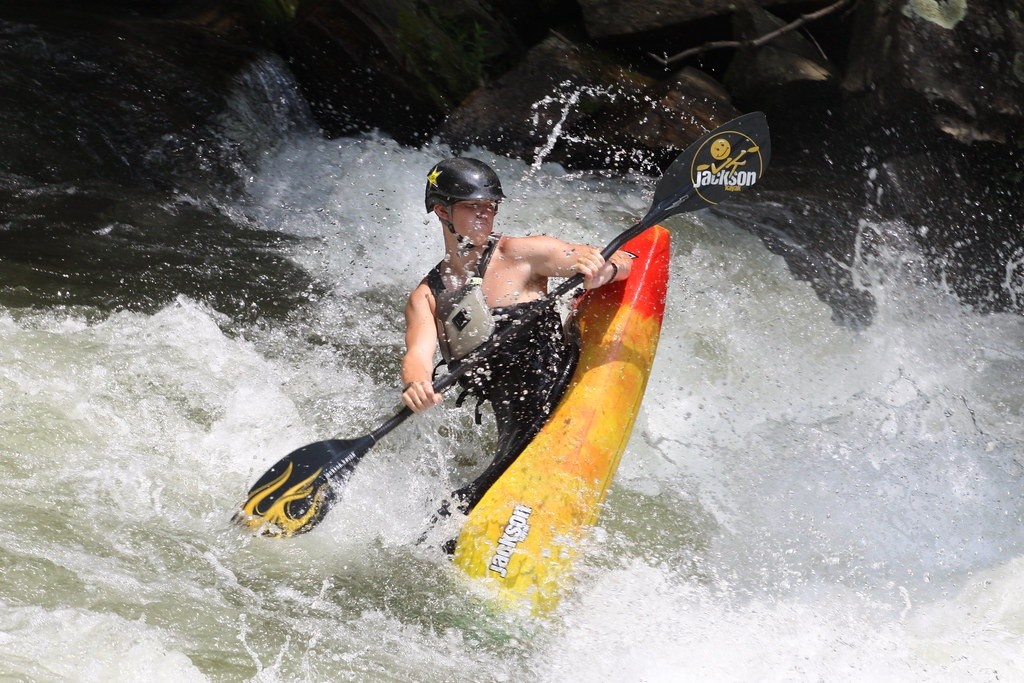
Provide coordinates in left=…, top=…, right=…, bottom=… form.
left=609, top=259, right=618, bottom=283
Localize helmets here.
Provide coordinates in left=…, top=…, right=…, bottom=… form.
left=425, top=157, right=507, bottom=213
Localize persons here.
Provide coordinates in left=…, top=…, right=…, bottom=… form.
left=402, top=158, right=633, bottom=554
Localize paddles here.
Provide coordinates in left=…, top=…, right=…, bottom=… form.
left=236, top=110, right=774, bottom=538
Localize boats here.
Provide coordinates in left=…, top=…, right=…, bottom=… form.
left=455, top=220, right=673, bottom=632
